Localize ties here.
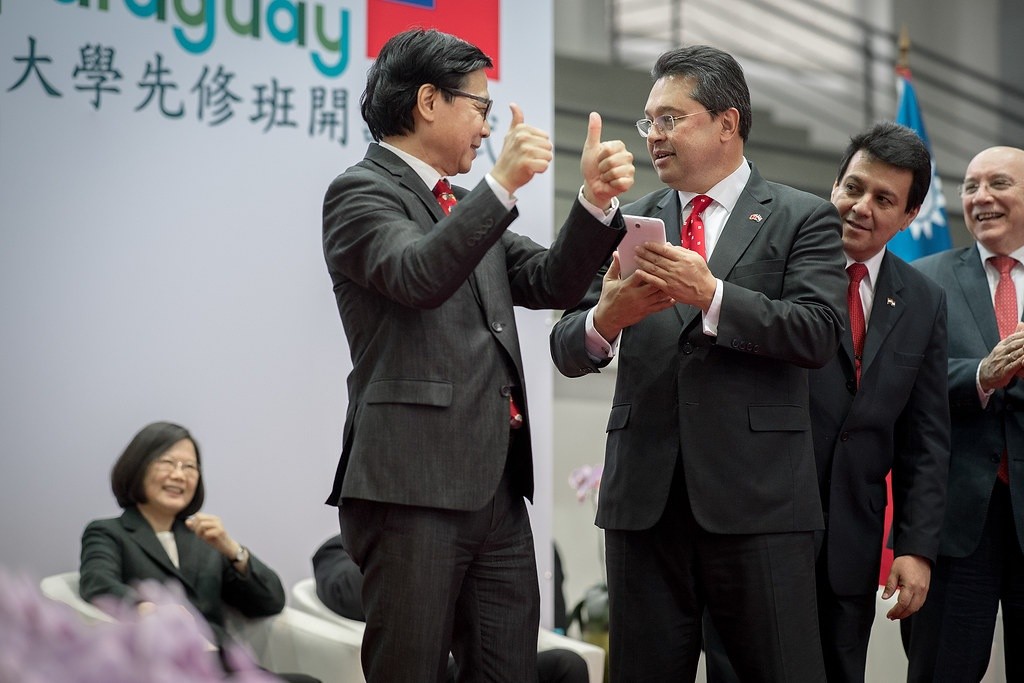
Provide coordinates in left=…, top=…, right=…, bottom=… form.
left=988, top=256, right=1018, bottom=484
left=432, top=180, right=524, bottom=428
left=681, top=194, right=713, bottom=263
left=846, top=264, right=866, bottom=393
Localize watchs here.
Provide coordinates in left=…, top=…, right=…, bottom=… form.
left=229, top=545, right=247, bottom=562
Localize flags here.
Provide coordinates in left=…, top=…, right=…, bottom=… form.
left=886, top=72, right=953, bottom=263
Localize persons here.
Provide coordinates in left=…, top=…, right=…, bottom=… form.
left=549, top=45, right=849, bottom=683
left=311, top=29, right=635, bottom=683
left=77, top=422, right=321, bottom=683
left=805, top=122, right=1024, bottom=682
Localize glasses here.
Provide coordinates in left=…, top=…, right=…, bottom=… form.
left=153, top=455, right=198, bottom=477
left=634, top=108, right=719, bottom=137
left=958, top=177, right=1024, bottom=198
left=439, top=87, right=494, bottom=121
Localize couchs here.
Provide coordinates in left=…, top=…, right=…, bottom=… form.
left=41, top=570, right=363, bottom=683
left=290, top=577, right=603, bottom=683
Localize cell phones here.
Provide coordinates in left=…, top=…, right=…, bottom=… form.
left=616, top=214, right=667, bottom=286
